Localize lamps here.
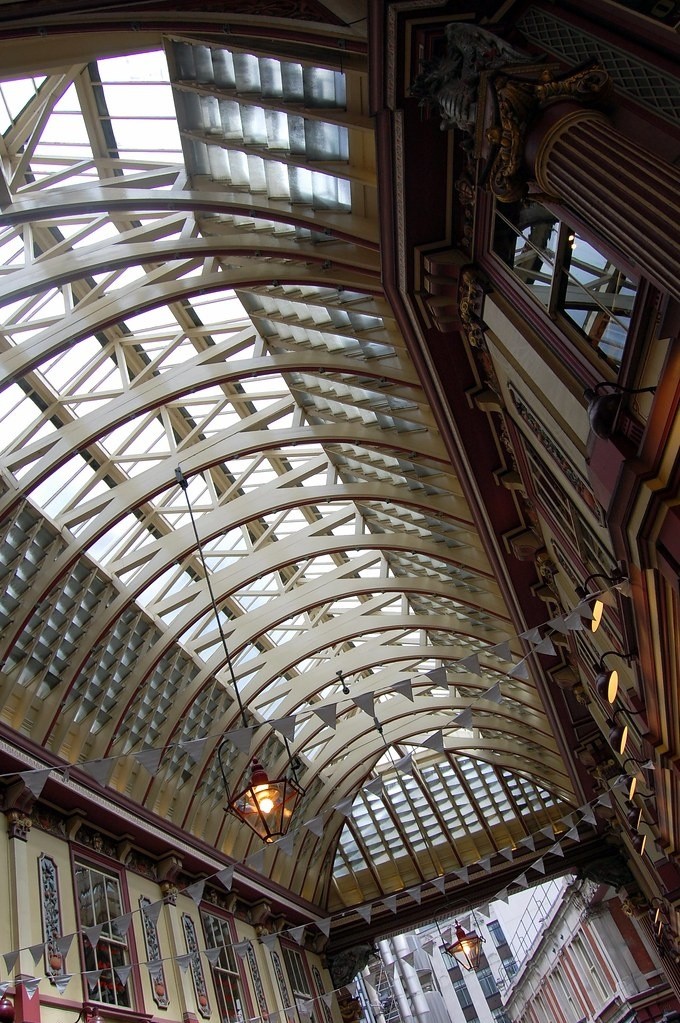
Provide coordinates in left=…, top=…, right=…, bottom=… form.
left=619, top=759, right=650, bottom=801
left=651, top=933, right=672, bottom=959
left=173, top=466, right=304, bottom=844
left=75, top=1007, right=106, bottom=1023
left=0, top=992, right=14, bottom=1023
left=378, top=727, right=486, bottom=972
left=581, top=382, right=659, bottom=439
left=606, top=709, right=646, bottom=755
left=626, top=821, right=658, bottom=856
left=651, top=919, right=671, bottom=939
left=573, top=567, right=623, bottom=633
left=624, top=792, right=656, bottom=829
left=593, top=651, right=638, bottom=704
left=649, top=897, right=669, bottom=923
